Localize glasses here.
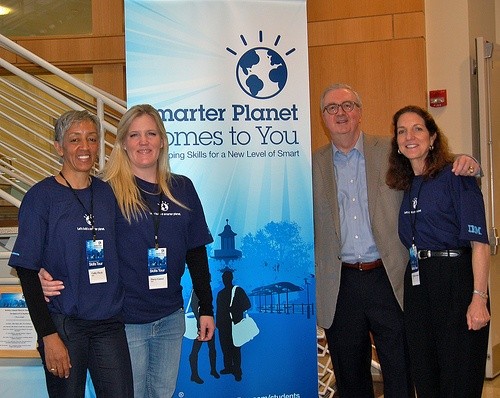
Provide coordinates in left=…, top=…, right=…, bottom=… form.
left=323, top=101, right=359, bottom=115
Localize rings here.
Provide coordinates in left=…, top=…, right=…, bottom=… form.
left=50, top=368, right=56, bottom=370
left=469, top=166, right=473, bottom=174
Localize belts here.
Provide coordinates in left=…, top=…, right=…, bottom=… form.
left=417, top=250, right=463, bottom=259
left=342, top=260, right=383, bottom=271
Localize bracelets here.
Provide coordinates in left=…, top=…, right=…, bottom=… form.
left=472, top=290, right=488, bottom=300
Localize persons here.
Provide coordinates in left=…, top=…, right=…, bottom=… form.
left=312, top=84, right=484, bottom=398
left=385, top=105, right=491, bottom=398
left=38, top=104, right=217, bottom=398
left=7, top=110, right=134, bottom=398
